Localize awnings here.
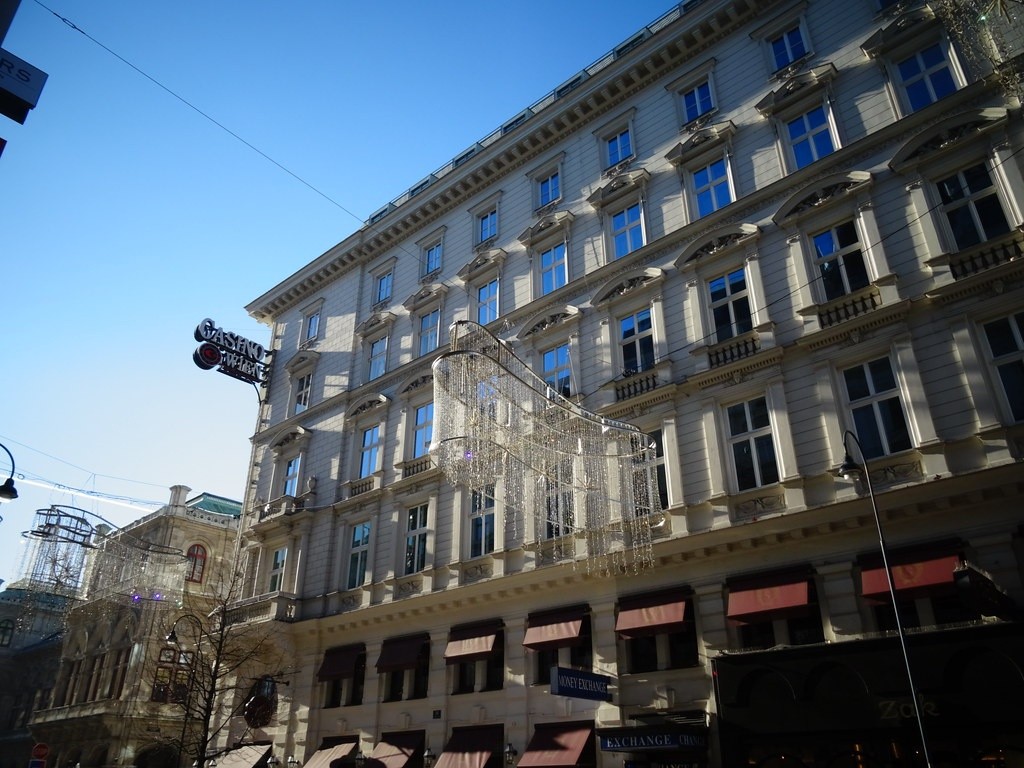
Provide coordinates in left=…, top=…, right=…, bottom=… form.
left=317, top=536, right=969, bottom=680
left=214, top=719, right=593, bottom=768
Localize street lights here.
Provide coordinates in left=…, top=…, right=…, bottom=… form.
left=826, top=429, right=932, bottom=768
left=166, top=615, right=203, bottom=768
